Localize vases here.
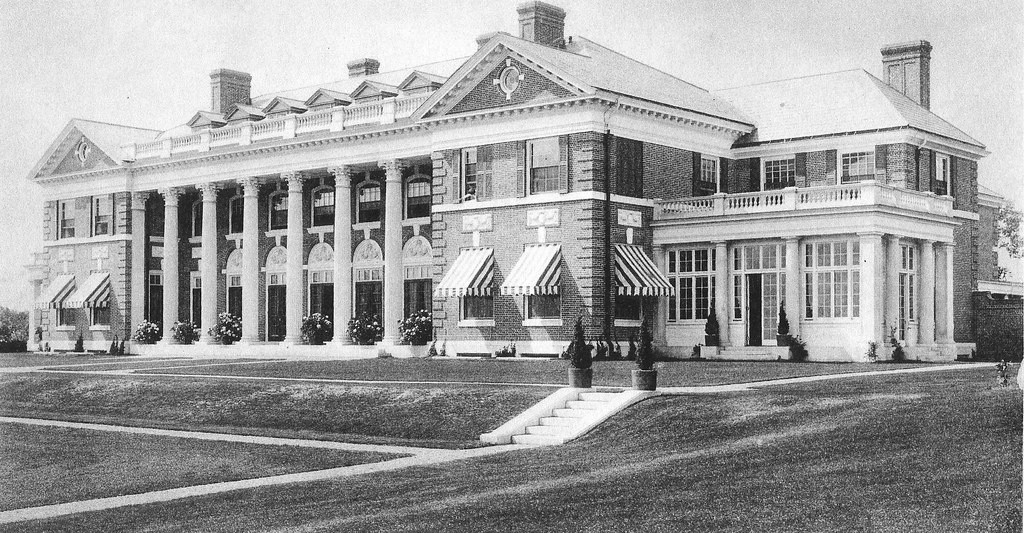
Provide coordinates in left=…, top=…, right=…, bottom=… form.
left=309, top=338, right=324, bottom=345
left=360, top=338, right=374, bottom=345
left=412, top=337, right=428, bottom=345
left=179, top=339, right=191, bottom=344
left=223, top=339, right=232, bottom=345
left=145, top=339, right=154, bottom=344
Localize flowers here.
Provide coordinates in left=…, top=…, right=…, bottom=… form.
left=207, top=311, right=242, bottom=345
left=135, top=319, right=161, bottom=342
left=397, top=309, right=432, bottom=346
left=170, top=320, right=200, bottom=344
left=300, top=313, right=334, bottom=344
left=346, top=312, right=385, bottom=345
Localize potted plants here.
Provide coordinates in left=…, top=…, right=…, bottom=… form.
left=631, top=310, right=658, bottom=391
left=705, top=299, right=720, bottom=346
left=776, top=300, right=792, bottom=346
left=567, top=317, right=593, bottom=388
